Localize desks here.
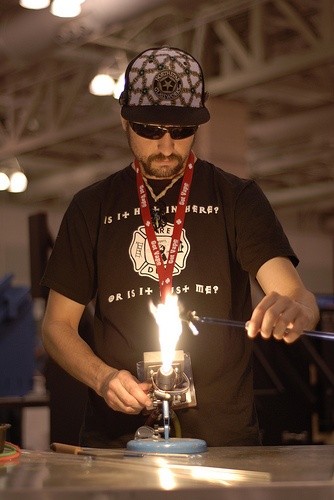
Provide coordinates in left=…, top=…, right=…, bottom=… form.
left=0, top=446, right=334, bottom=500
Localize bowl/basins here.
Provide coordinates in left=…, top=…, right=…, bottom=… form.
left=0, top=424, right=11, bottom=453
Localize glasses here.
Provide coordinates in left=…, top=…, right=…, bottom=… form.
left=129, top=121, right=198, bottom=140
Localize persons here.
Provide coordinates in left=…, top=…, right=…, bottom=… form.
left=39, top=44, right=320, bottom=449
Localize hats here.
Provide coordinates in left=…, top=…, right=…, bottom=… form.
left=119, top=47, right=211, bottom=127
